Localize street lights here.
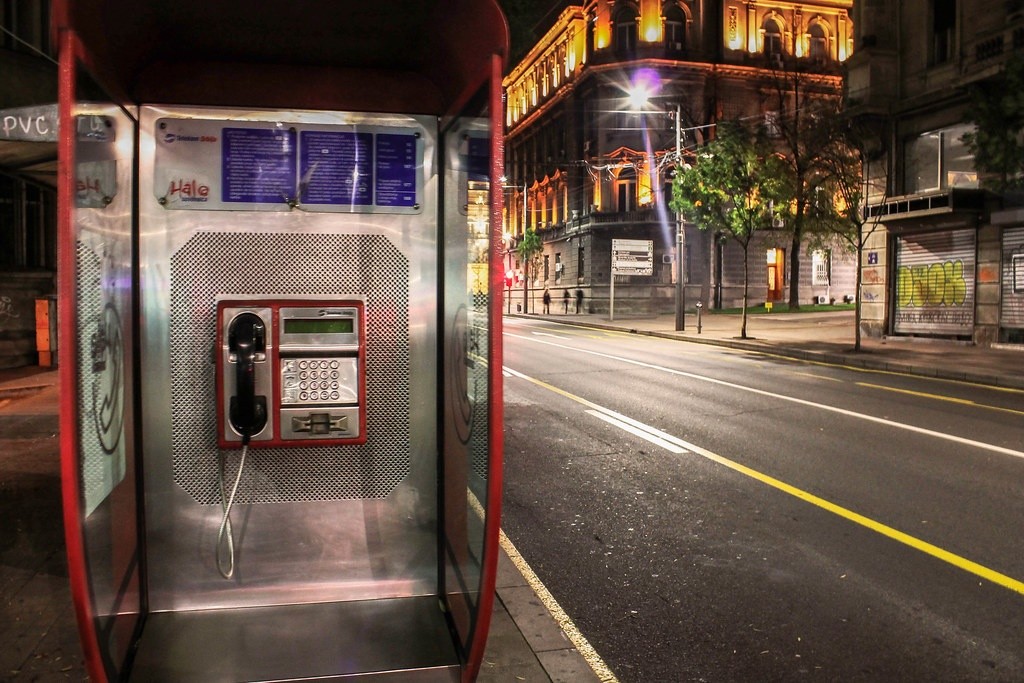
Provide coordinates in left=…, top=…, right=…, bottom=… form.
left=504, top=233, right=511, bottom=314
left=628, top=89, right=686, bottom=331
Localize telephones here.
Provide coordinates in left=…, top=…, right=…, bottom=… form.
left=214, top=291, right=368, bottom=449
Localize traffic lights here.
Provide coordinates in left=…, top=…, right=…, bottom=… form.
left=505, top=270, right=514, bottom=287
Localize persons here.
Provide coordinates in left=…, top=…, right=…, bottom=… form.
left=561, top=288, right=571, bottom=314
left=575, top=286, right=584, bottom=314
left=542, top=288, right=551, bottom=314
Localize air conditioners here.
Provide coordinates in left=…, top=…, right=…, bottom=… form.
left=556, top=262, right=561, bottom=271
left=818, top=295, right=829, bottom=304
left=847, top=294, right=854, bottom=302
